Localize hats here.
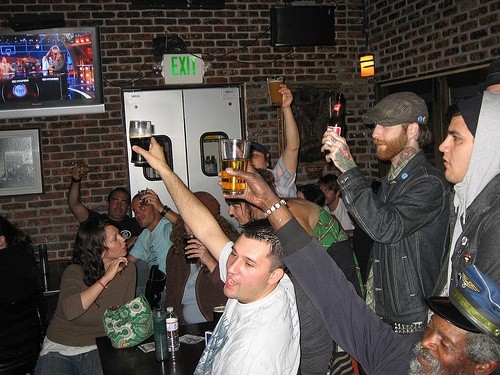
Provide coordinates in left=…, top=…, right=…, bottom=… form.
left=478, top=59, right=500, bottom=94
left=424, top=263, right=500, bottom=338
left=250, top=142, right=270, bottom=156
left=361, top=91, right=428, bottom=125
left=193, top=192, right=221, bottom=214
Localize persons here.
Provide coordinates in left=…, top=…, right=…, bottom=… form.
left=132, top=138, right=300, bottom=375
left=35, top=215, right=137, bottom=374
left=290, top=56, right=500, bottom=375
left=217, top=143, right=500, bottom=375
left=0, top=214, right=40, bottom=362
left=0, top=52, right=67, bottom=77
left=69, top=166, right=142, bottom=253
left=161, top=192, right=241, bottom=325
left=220, top=168, right=362, bottom=297
left=86, top=47, right=93, bottom=59
left=126, top=187, right=178, bottom=310
left=239, top=83, right=302, bottom=197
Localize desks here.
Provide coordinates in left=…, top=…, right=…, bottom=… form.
left=95, top=320, right=219, bottom=375
left=37, top=260, right=70, bottom=297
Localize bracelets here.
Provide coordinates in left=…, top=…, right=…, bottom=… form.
left=264, top=200, right=287, bottom=218
left=98, top=280, right=106, bottom=289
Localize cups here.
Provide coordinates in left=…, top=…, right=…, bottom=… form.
left=128, top=121, right=152, bottom=164
left=213, top=304, right=226, bottom=328
left=218, top=138, right=250, bottom=196
left=138, top=190, right=148, bottom=205
left=184, top=235, right=198, bottom=264
left=78, top=164, right=87, bottom=178
left=267, top=76, right=284, bottom=106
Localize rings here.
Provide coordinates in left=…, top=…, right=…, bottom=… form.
left=196, top=244, right=201, bottom=248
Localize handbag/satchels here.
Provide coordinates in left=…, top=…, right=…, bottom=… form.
left=102, top=296, right=157, bottom=349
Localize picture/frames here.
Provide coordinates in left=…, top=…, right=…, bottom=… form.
left=277, top=82, right=343, bottom=168
left=0, top=129, right=45, bottom=196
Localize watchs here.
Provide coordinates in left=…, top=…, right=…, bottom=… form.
left=71, top=175, right=82, bottom=183
left=160, top=205, right=170, bottom=217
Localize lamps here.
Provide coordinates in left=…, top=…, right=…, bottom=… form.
left=359, top=16, right=374, bottom=77
left=153, top=53, right=212, bottom=85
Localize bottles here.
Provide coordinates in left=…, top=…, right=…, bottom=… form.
left=165, top=307, right=180, bottom=352
left=326, top=92, right=346, bottom=164
left=153, top=309, right=168, bottom=361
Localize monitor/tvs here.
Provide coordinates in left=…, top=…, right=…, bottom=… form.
left=0, top=25, right=103, bottom=110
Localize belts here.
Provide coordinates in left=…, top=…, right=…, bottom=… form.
left=386, top=320, right=427, bottom=334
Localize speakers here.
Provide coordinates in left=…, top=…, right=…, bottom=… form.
left=271, top=5, right=337, bottom=46
